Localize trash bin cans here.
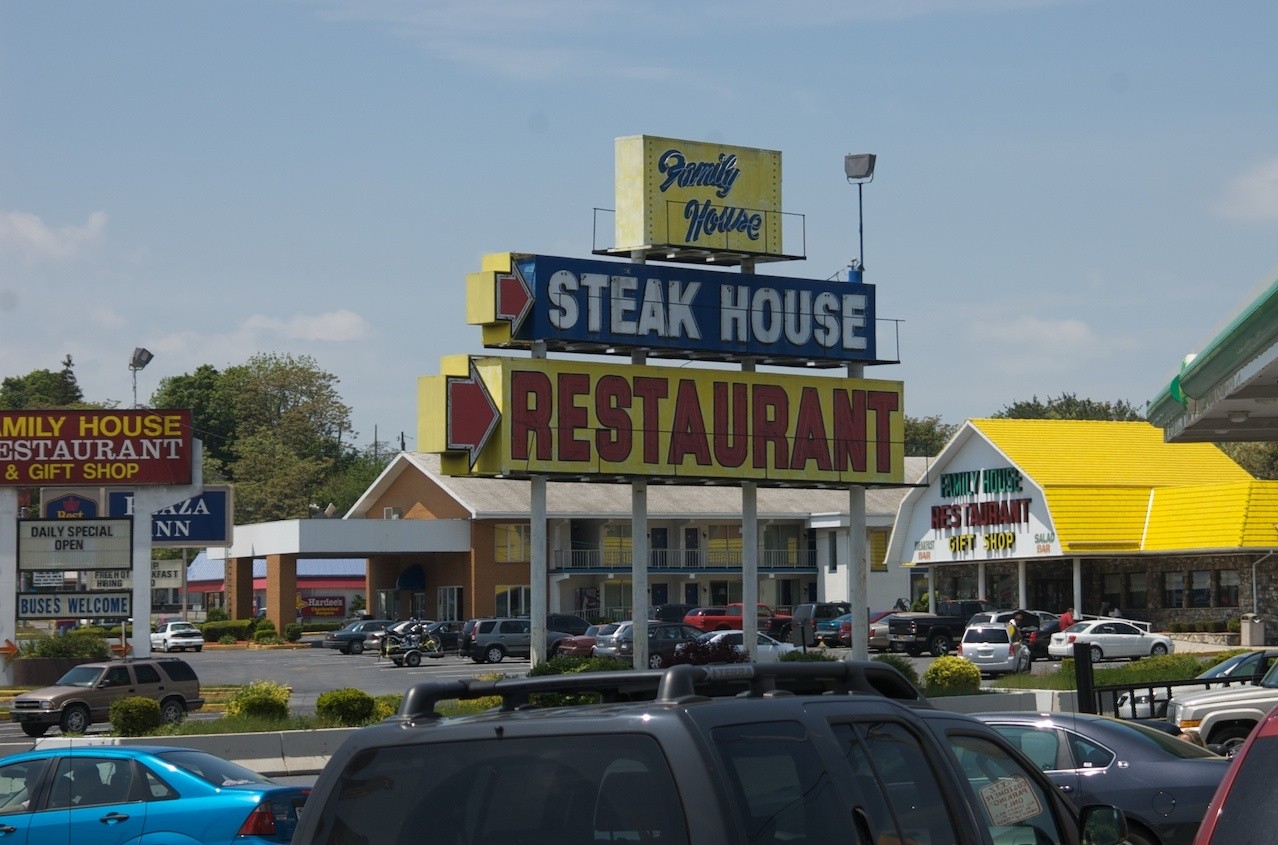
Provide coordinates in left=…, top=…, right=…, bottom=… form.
left=1239, top=612, right=1268, bottom=646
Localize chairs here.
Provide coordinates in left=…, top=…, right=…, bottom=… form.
left=111, top=768, right=142, bottom=801
left=51, top=770, right=77, bottom=808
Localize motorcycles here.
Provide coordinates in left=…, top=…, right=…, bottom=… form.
left=379, top=617, right=443, bottom=667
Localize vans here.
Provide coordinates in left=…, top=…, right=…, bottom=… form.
left=656, top=604, right=726, bottom=622
left=792, top=602, right=851, bottom=647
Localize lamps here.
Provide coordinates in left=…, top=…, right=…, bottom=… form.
left=128, top=348, right=155, bottom=371
left=844, top=154, right=876, bottom=184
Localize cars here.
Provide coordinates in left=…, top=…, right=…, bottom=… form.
left=953, top=650, right=1278, bottom=845
left=0, top=746, right=315, bottom=845
left=555, top=619, right=805, bottom=669
left=813, top=610, right=894, bottom=650
left=152, top=620, right=205, bottom=652
left=324, top=618, right=464, bottom=655
left=956, top=608, right=1176, bottom=675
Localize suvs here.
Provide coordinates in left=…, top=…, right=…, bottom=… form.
left=293, top=661, right=1129, bottom=845
left=8, top=657, right=207, bottom=737
left=464, top=613, right=592, bottom=664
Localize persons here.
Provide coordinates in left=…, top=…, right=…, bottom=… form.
left=1009, top=611, right=1023, bottom=638
left=1128, top=720, right=1207, bottom=748
left=72, top=762, right=110, bottom=807
left=1059, top=608, right=1077, bottom=632
left=0, top=763, right=54, bottom=814
left=1107, top=605, right=1122, bottom=618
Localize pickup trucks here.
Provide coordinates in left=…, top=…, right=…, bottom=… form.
left=685, top=602, right=794, bottom=643
left=888, top=599, right=1000, bottom=659
